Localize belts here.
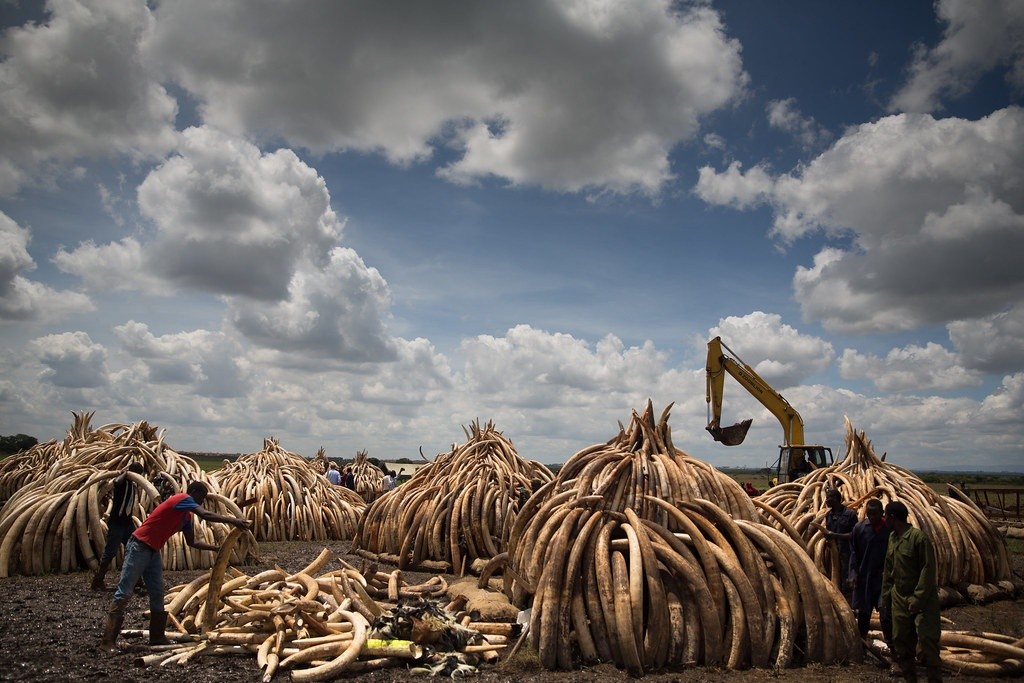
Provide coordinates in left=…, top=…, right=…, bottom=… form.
left=130, top=535, right=155, bottom=552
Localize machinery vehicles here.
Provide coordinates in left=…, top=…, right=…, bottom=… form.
left=705, top=336, right=833, bottom=485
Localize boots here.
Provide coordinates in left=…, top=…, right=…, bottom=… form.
left=100, top=613, right=124, bottom=655
left=149, top=612, right=171, bottom=645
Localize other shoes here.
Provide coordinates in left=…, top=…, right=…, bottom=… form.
left=92, top=571, right=109, bottom=591
left=135, top=576, right=146, bottom=590
left=888, top=644, right=904, bottom=675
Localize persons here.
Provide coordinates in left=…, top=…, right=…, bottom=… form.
left=92, top=461, right=145, bottom=589
left=878, top=500, right=942, bottom=683
left=848, top=499, right=904, bottom=676
left=822, top=490, right=860, bottom=605
left=742, top=478, right=780, bottom=497
left=948, top=480, right=971, bottom=505
left=322, top=462, right=404, bottom=497
left=98, top=479, right=251, bottom=655
